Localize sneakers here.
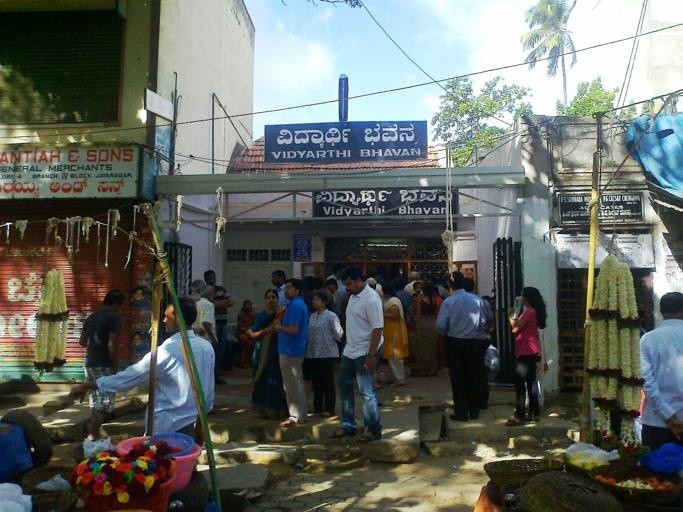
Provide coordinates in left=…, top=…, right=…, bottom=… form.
left=308, top=409, right=320, bottom=413
left=525, top=414, right=540, bottom=421
left=329, top=428, right=356, bottom=438
left=506, top=418, right=525, bottom=425
left=356, top=432, right=381, bottom=443
left=320, top=411, right=336, bottom=417
left=279, top=419, right=294, bottom=427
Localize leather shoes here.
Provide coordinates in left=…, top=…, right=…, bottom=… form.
left=451, top=415, right=467, bottom=421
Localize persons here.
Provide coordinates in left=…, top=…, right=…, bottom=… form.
left=70, top=269, right=233, bottom=445
left=506, top=286, right=547, bottom=427
left=236, top=265, right=494, bottom=444
left=639, top=292, right=683, bottom=451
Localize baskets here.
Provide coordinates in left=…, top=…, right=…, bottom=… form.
left=588, top=464, right=683, bottom=504
left=517, top=470, right=624, bottom=512
left=22, top=466, right=80, bottom=512
left=483, top=459, right=563, bottom=504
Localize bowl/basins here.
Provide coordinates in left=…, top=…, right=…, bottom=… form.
left=151, top=432, right=193, bottom=456
left=116, top=435, right=201, bottom=490
